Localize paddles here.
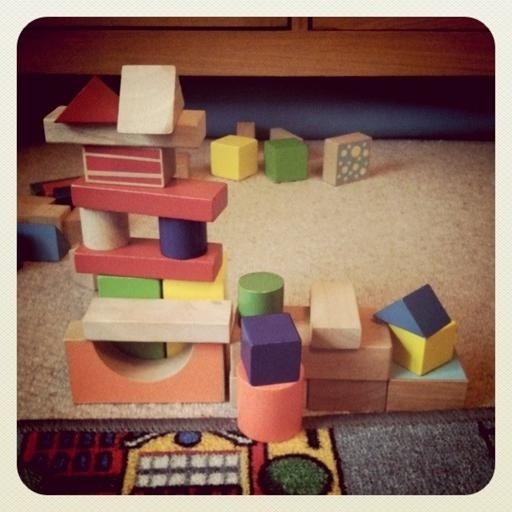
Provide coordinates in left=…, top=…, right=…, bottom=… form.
left=16, top=404, right=494, bottom=495
